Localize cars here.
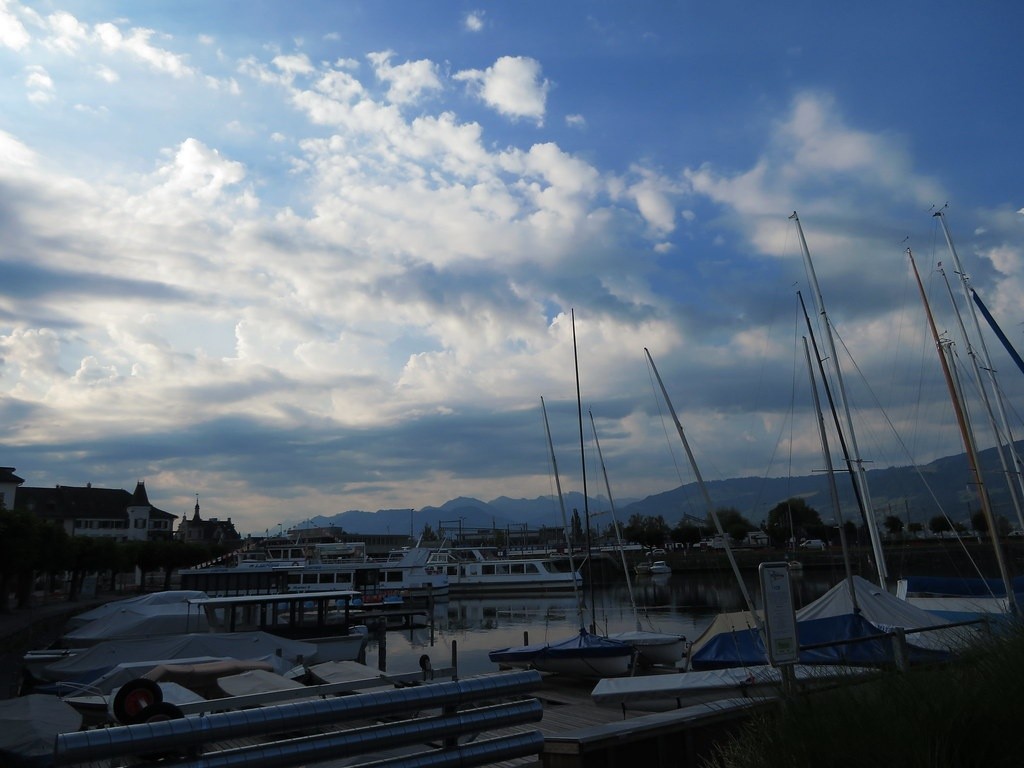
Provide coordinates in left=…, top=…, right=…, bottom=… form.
left=645, top=549, right=667, bottom=556
left=634, top=562, right=651, bottom=574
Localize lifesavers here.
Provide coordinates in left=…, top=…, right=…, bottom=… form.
left=133, top=701, right=185, bottom=725
left=292, top=562, right=299, bottom=566
left=113, top=678, right=163, bottom=725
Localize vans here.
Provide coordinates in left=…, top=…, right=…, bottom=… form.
left=800, top=539, right=823, bottom=550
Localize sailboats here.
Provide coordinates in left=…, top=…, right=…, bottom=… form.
left=486, top=201, right=1024, bottom=694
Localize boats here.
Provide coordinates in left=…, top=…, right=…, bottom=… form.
left=178, top=542, right=582, bottom=606
left=21, top=591, right=365, bottom=684
left=649, top=561, right=672, bottom=574
